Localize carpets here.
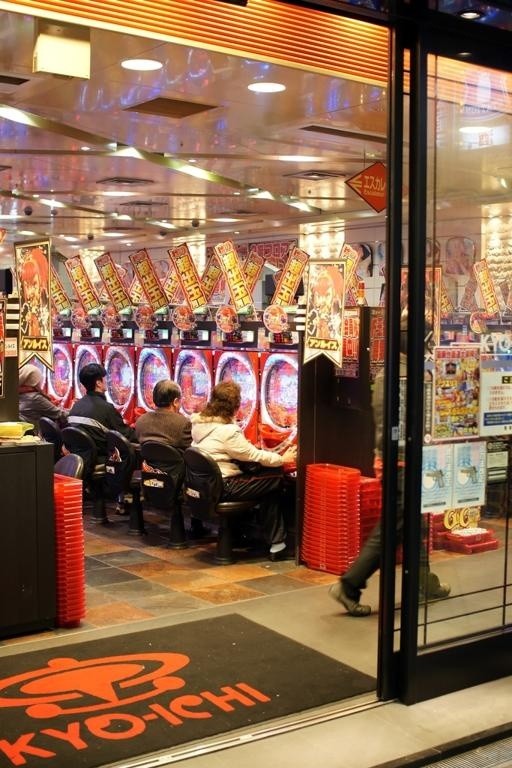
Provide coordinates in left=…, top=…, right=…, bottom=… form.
left=2, top=613, right=383, bottom=768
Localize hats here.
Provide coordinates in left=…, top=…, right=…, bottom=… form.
left=18, top=364, right=42, bottom=387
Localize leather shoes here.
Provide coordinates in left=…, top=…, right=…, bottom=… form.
left=269, top=542, right=295, bottom=561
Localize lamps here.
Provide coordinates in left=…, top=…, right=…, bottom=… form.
left=31, top=15, right=93, bottom=83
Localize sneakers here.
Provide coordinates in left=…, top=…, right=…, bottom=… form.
left=116, top=503, right=129, bottom=514
left=328, top=582, right=371, bottom=616
left=187, top=528, right=219, bottom=537
left=419, top=583, right=451, bottom=602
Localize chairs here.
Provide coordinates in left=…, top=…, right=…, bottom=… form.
left=33, top=415, right=286, bottom=564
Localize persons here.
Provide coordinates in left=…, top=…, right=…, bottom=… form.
left=328, top=321, right=451, bottom=616
left=136, top=379, right=213, bottom=538
left=68, top=364, right=139, bottom=515
left=189, top=379, right=296, bottom=560
left=19, top=364, right=69, bottom=462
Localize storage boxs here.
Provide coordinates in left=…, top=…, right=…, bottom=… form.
left=297, top=459, right=499, bottom=575
left=49, top=469, right=86, bottom=629
